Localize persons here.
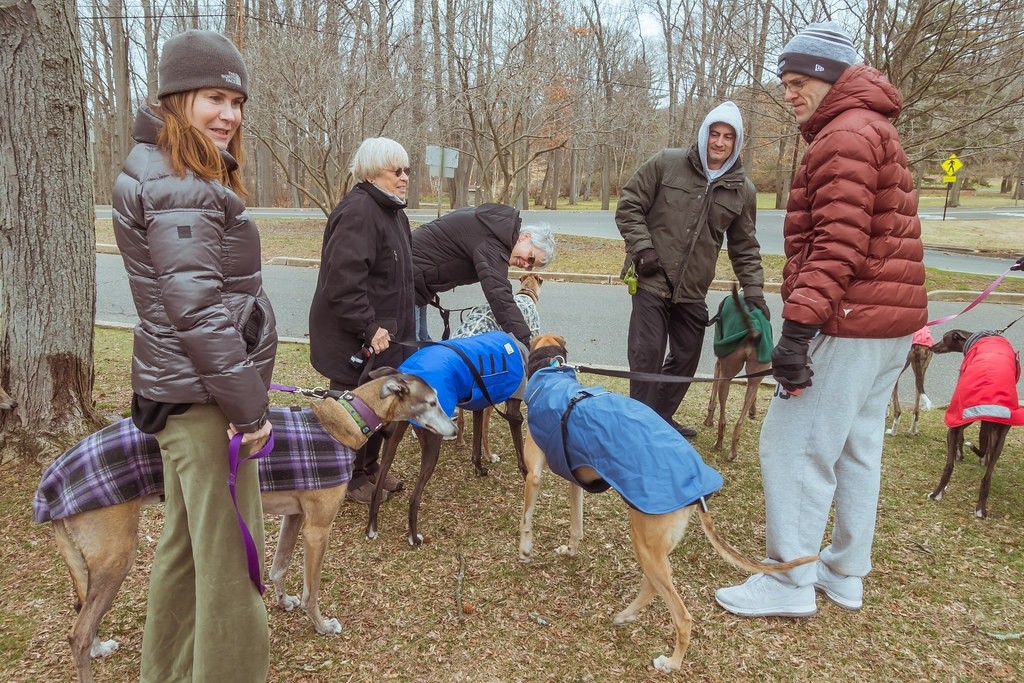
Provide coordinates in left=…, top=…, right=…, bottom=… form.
left=715, top=22, right=928, bottom=617
left=616, top=101, right=772, bottom=437
left=309, top=137, right=416, bottom=504
left=111, top=29, right=279, bottom=683
left=409, top=202, right=555, bottom=440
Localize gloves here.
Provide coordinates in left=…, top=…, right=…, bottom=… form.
left=770, top=335, right=814, bottom=393
left=745, top=295, right=770, bottom=321
left=633, top=248, right=660, bottom=279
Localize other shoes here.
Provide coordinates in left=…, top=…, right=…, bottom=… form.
left=669, top=418, right=698, bottom=438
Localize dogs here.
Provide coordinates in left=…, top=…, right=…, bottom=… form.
left=519, top=334, right=819, bottom=673
left=703, top=283, right=774, bottom=462
left=930, top=330, right=1017, bottom=519
left=452, top=273, right=543, bottom=463
left=885, top=325, right=934, bottom=436
left=51, top=365, right=461, bottom=683
left=364, top=331, right=528, bottom=545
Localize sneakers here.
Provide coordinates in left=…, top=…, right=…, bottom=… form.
left=344, top=481, right=391, bottom=505
left=715, top=570, right=817, bottom=617
left=813, top=559, right=862, bottom=610
left=365, top=462, right=405, bottom=491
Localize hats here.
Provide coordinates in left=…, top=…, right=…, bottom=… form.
left=776, top=22, right=857, bottom=84
left=157, top=29, right=249, bottom=103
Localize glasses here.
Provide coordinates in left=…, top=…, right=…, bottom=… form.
left=383, top=166, right=411, bottom=177
left=782, top=74, right=814, bottom=93
left=526, top=239, right=536, bottom=271
